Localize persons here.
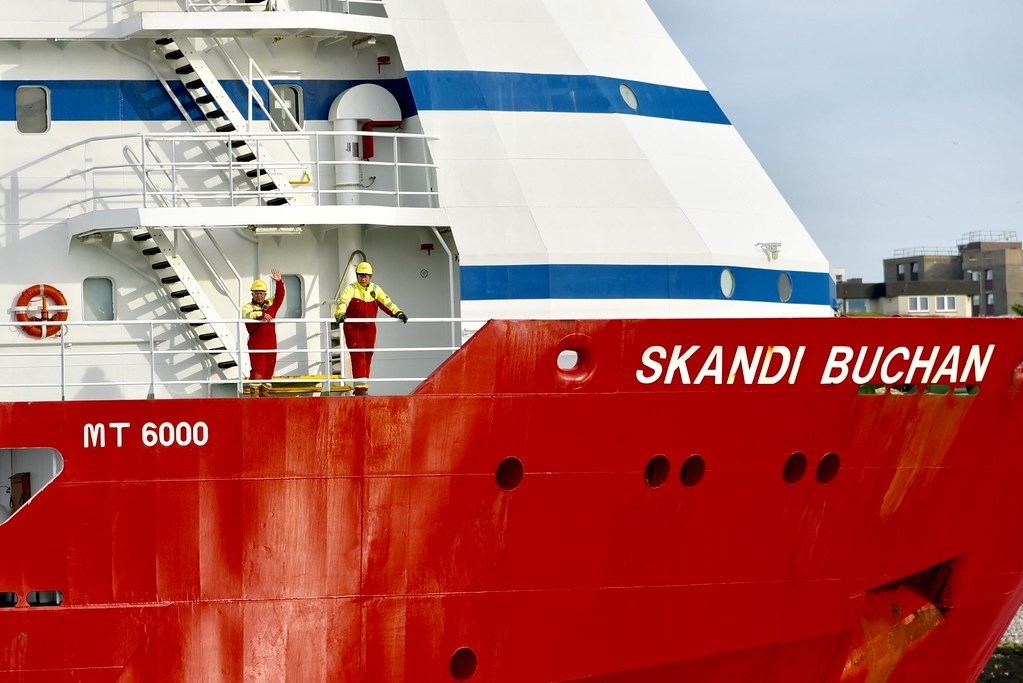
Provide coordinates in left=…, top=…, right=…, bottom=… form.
left=334, top=261, right=407, bottom=397
left=242, top=268, right=286, bottom=396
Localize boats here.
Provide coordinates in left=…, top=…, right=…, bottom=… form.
left=0, top=0, right=1023, bottom=683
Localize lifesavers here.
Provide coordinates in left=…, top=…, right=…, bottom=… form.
left=16, top=284, right=68, bottom=338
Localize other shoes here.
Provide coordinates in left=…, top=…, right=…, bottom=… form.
left=357, top=390, right=369, bottom=396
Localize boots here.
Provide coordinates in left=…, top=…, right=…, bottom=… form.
left=260, top=384, right=272, bottom=398
left=250, top=383, right=261, bottom=397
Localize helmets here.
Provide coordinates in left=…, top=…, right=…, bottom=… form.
left=355, top=262, right=372, bottom=275
left=250, top=280, right=267, bottom=292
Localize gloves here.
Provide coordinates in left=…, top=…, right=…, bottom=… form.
left=398, top=313, right=408, bottom=324
left=336, top=314, right=346, bottom=323
